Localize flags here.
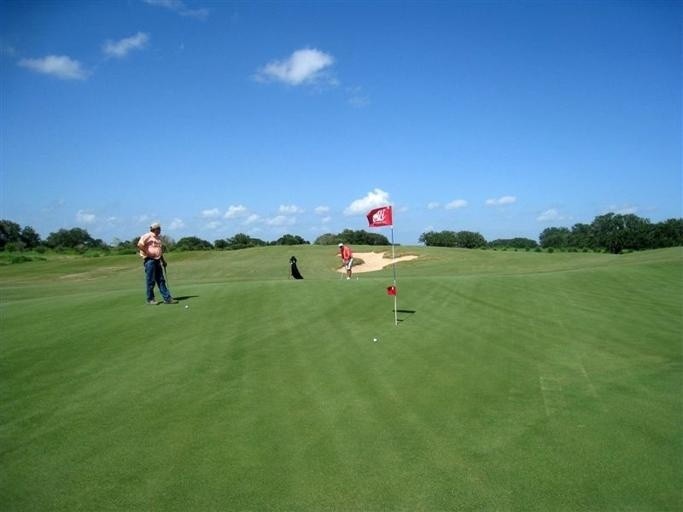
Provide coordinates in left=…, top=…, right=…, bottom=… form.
left=366, top=206, right=394, bottom=227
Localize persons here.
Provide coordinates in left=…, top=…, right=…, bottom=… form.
left=137, top=223, right=179, bottom=305
left=335, top=242, right=354, bottom=280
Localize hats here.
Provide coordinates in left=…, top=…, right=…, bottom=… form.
left=150, top=223, right=159, bottom=230
left=338, top=243, right=344, bottom=248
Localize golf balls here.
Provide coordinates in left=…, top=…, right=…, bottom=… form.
left=184, top=306, right=189, bottom=309
left=374, top=339, right=377, bottom=342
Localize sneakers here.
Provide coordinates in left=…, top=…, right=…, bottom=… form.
left=147, top=300, right=159, bottom=305
left=164, top=299, right=178, bottom=304
